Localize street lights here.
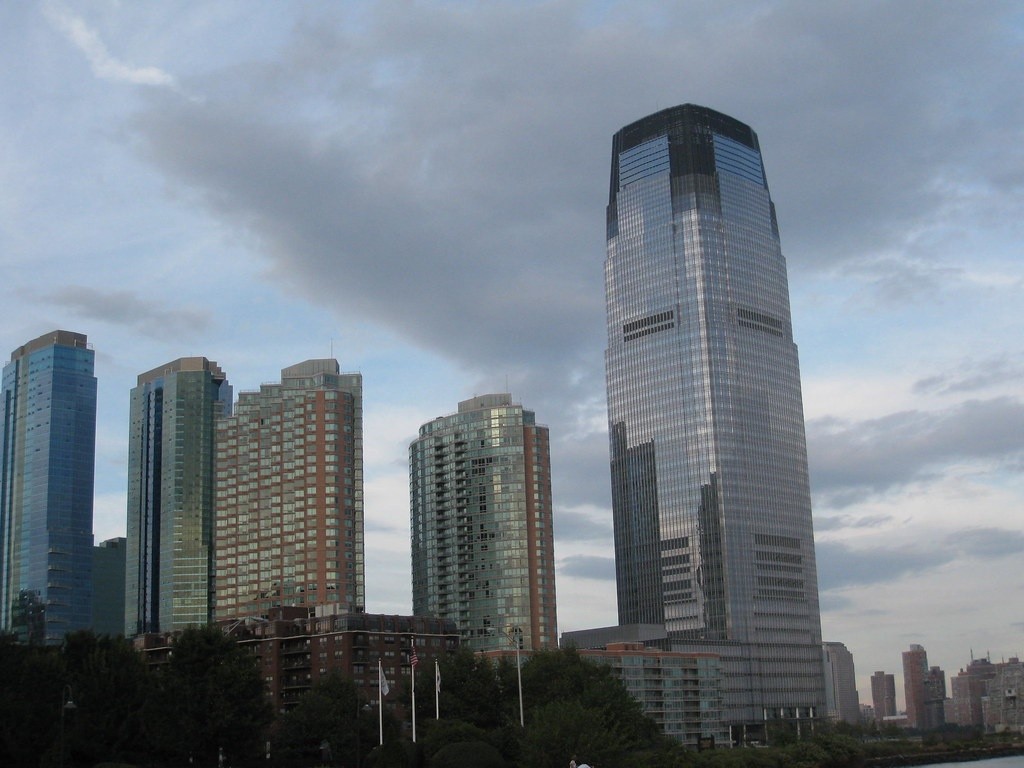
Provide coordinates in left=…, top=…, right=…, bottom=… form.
left=60, top=685, right=77, bottom=767
left=487, top=628, right=524, bottom=727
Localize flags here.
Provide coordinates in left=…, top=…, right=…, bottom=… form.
left=437, top=664, right=441, bottom=693
left=381, top=666, right=389, bottom=696
left=410, top=645, right=419, bottom=669
